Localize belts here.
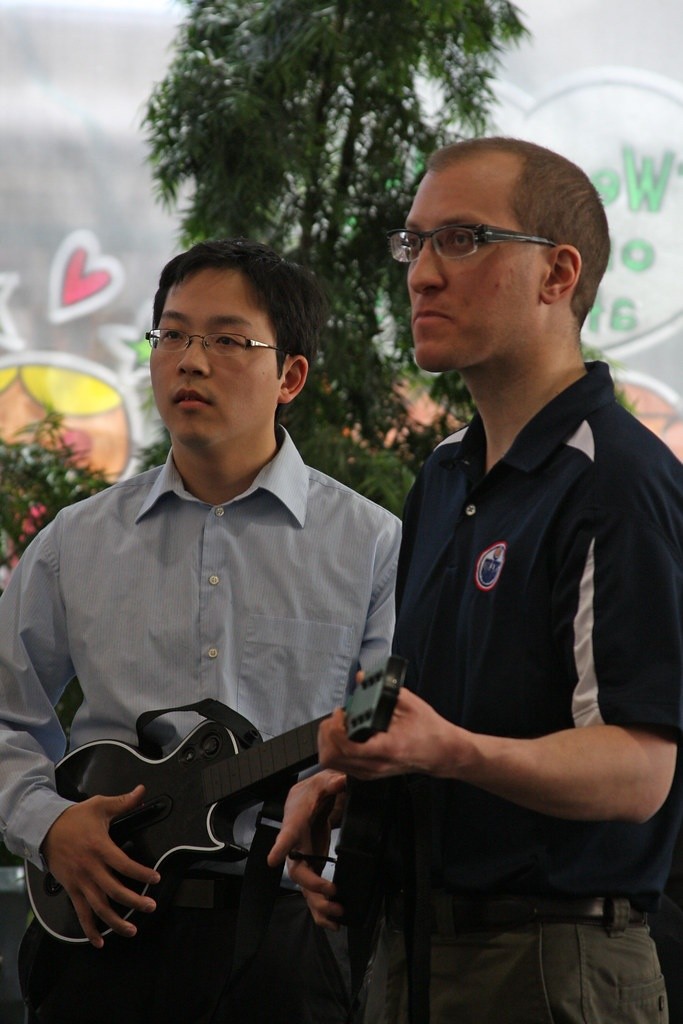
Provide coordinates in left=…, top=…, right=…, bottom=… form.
left=166, top=875, right=301, bottom=911
left=383, top=888, right=644, bottom=935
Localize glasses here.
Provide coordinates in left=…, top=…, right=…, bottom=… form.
left=146, top=328, right=292, bottom=357
left=385, top=224, right=556, bottom=262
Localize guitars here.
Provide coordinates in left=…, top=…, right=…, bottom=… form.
left=25, top=700, right=337, bottom=944
left=315, top=652, right=410, bottom=957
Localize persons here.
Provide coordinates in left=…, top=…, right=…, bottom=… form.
left=0, top=238, right=404, bottom=1024
left=266, top=138, right=683, bottom=1024
